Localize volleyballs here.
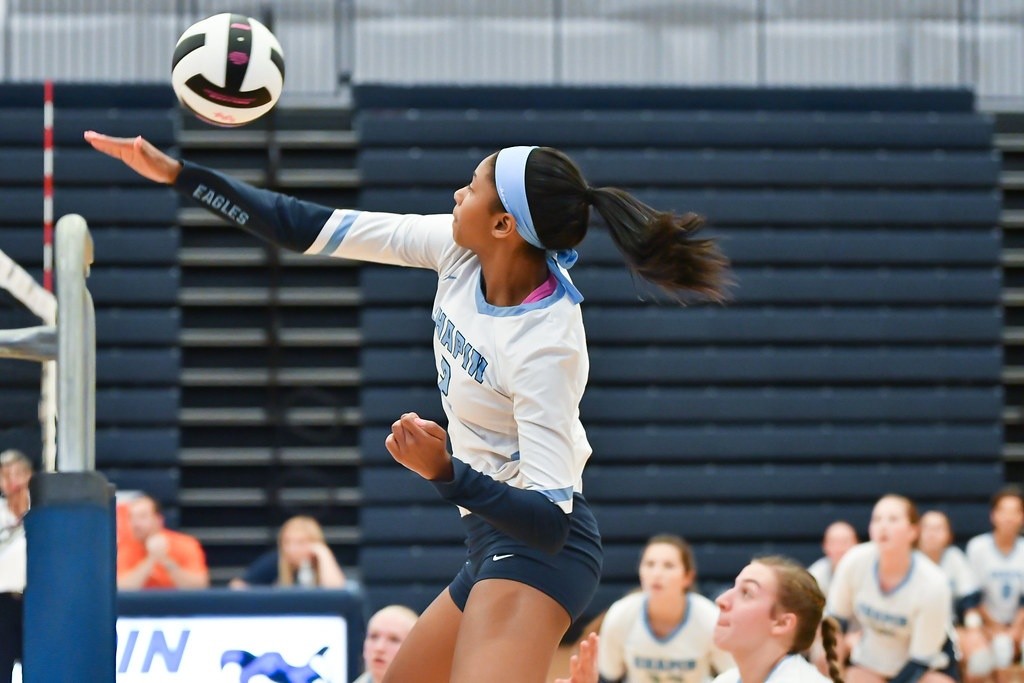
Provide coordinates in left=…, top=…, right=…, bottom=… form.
left=170, top=11, right=288, bottom=132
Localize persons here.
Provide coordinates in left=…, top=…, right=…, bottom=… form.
left=84, top=130, right=723, bottom=683
left=353, top=606, right=418, bottom=683
left=228, top=516, right=348, bottom=590
left=555, top=534, right=844, bottom=683
left=116, top=496, right=208, bottom=590
left=0, top=450, right=33, bottom=683
left=808, top=488, right=1024, bottom=683
